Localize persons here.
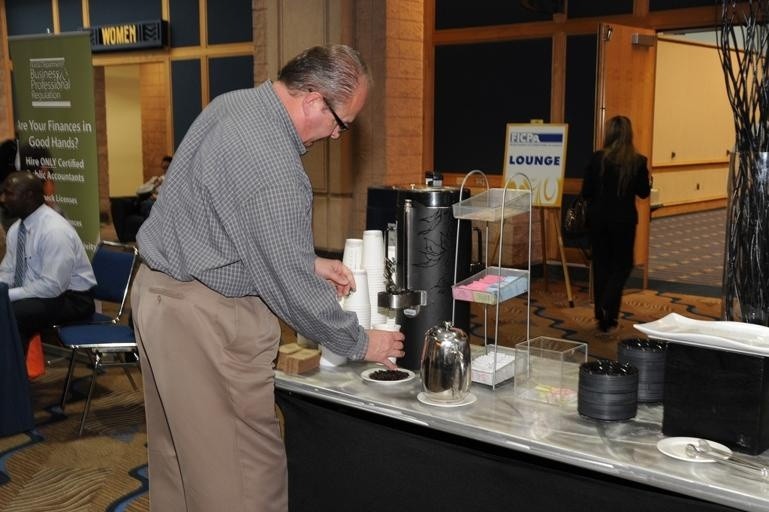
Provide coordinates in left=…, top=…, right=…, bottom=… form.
left=582, top=115, right=651, bottom=337
left=137, top=156, right=171, bottom=201
left=130, top=41, right=373, bottom=512
left=0, top=171, right=98, bottom=359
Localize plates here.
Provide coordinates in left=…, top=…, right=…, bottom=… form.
left=360, top=368, right=415, bottom=386
left=417, top=390, right=477, bottom=408
left=634, top=311, right=769, bottom=354
left=656, top=437, right=732, bottom=462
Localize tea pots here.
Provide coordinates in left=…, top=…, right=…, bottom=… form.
left=420, top=321, right=472, bottom=403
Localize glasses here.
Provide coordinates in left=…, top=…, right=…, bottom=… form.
left=162, top=166, right=168, bottom=170
left=309, top=89, right=348, bottom=134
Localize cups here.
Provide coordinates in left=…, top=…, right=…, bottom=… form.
left=372, top=325, right=401, bottom=365
left=324, top=230, right=396, bottom=368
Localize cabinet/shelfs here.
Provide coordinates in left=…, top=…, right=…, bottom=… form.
left=449, top=168, right=532, bottom=393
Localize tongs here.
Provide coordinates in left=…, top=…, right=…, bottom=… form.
left=685, top=440, right=767, bottom=478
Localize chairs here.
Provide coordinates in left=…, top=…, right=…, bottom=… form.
left=41, top=239, right=139, bottom=392
left=56, top=309, right=157, bottom=436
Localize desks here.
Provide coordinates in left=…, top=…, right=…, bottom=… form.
left=274, top=326, right=769, bottom=512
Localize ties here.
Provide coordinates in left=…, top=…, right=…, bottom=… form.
left=13, top=222, right=26, bottom=288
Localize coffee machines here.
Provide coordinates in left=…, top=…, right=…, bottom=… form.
left=364, top=180, right=474, bottom=372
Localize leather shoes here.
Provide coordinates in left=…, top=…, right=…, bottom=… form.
left=597, top=320, right=619, bottom=333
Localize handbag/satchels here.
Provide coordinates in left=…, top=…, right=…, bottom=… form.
left=562, top=194, right=591, bottom=247
